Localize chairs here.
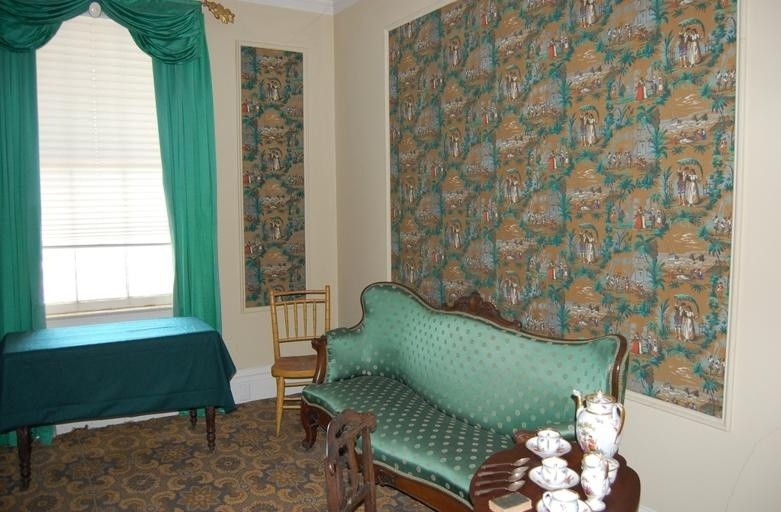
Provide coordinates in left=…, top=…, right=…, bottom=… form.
left=319, top=407, right=381, bottom=511
left=267, top=285, right=334, bottom=439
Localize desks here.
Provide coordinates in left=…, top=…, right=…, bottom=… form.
left=0, top=315, right=229, bottom=490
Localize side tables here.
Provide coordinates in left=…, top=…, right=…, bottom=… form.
left=470, top=433, right=641, bottom=511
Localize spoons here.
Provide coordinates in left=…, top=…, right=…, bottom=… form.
left=474, top=456, right=531, bottom=496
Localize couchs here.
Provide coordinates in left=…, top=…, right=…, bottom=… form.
left=299, top=280, right=631, bottom=511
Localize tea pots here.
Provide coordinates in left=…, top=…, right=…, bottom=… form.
left=571, top=389, right=626, bottom=458
left=578, top=450, right=611, bottom=512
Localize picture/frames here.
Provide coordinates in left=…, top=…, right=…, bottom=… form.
left=383, top=0, right=751, bottom=434
left=235, top=38, right=313, bottom=315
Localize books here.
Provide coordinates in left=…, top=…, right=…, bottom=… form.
left=489, top=492, right=534, bottom=512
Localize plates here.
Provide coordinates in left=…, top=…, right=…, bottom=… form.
left=529, top=465, right=580, bottom=491
left=536, top=497, right=592, bottom=512
left=525, top=436, right=572, bottom=458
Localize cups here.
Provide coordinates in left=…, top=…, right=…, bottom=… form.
left=541, top=458, right=568, bottom=484
left=541, top=489, right=580, bottom=512
left=580, top=459, right=620, bottom=485
left=536, top=430, right=560, bottom=453
